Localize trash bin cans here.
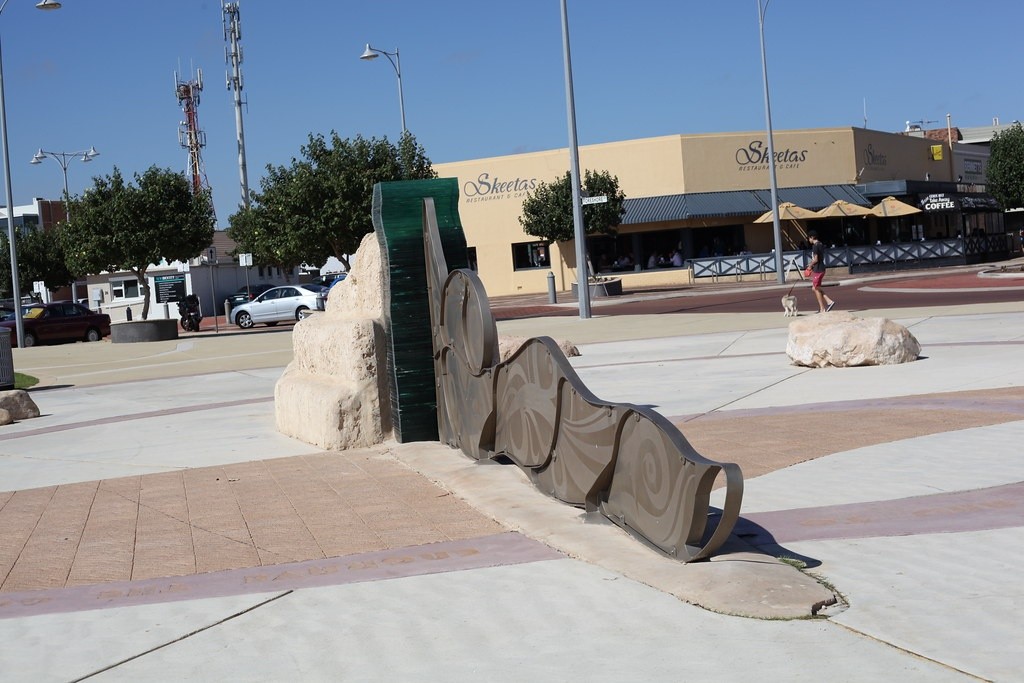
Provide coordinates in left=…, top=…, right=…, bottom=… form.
left=0, top=327, right=15, bottom=392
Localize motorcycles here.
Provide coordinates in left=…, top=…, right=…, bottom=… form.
left=176, top=296, right=203, bottom=332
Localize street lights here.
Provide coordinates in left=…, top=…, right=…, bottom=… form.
left=0, top=0, right=63, bottom=347
left=29, top=145, right=100, bottom=223
left=359, top=42, right=407, bottom=134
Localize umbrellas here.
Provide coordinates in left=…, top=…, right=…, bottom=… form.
left=863, top=196, right=923, bottom=243
left=752, top=202, right=827, bottom=251
left=816, top=199, right=877, bottom=247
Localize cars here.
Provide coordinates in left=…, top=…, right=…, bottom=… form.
left=312, top=273, right=347, bottom=287
left=0, top=298, right=89, bottom=321
left=229, top=284, right=291, bottom=309
left=0, top=302, right=112, bottom=347
left=231, top=281, right=329, bottom=329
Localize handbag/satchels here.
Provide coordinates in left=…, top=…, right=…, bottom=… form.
left=804, top=269, right=811, bottom=277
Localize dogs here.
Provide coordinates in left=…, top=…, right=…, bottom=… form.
left=781, top=294, right=798, bottom=318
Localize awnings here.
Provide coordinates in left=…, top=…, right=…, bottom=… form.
left=914, top=192, right=1005, bottom=214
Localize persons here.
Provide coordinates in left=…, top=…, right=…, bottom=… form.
left=796, top=241, right=807, bottom=251
left=936, top=227, right=986, bottom=240
left=597, top=242, right=752, bottom=273
left=806, top=230, right=835, bottom=314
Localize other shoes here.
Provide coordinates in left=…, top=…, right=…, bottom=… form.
left=826, top=301, right=835, bottom=312
